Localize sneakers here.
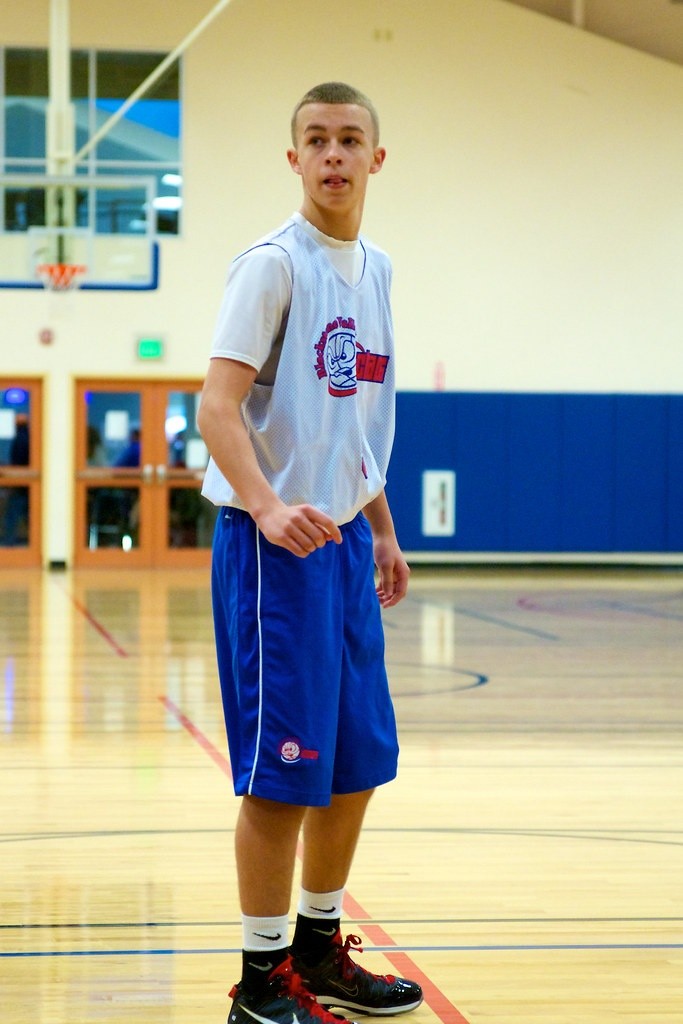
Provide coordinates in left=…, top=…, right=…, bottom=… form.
left=226, top=953, right=358, bottom=1024
left=293, top=934, right=424, bottom=1015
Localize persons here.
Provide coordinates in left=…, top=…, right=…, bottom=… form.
left=198, top=81, right=424, bottom=1024
